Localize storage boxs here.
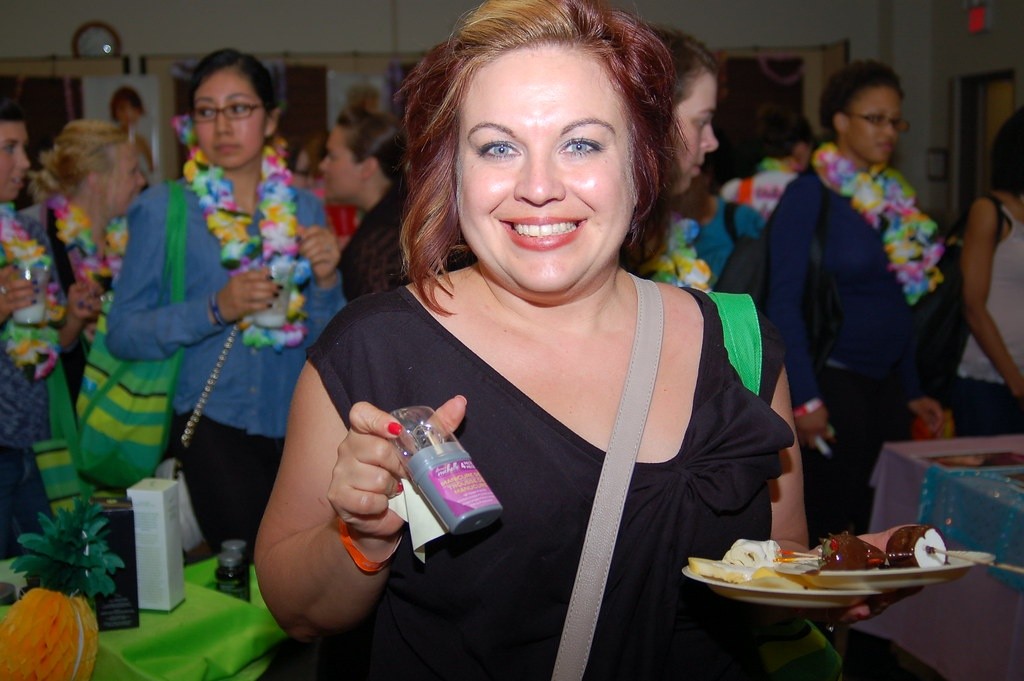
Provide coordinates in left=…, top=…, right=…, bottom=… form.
left=88, top=476, right=185, bottom=633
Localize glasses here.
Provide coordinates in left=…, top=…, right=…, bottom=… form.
left=850, top=113, right=910, bottom=132
left=190, top=102, right=264, bottom=123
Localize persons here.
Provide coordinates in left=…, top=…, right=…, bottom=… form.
left=255, top=0, right=809, bottom=681
left=720, top=110, right=817, bottom=222
left=285, top=140, right=313, bottom=188
left=620, top=22, right=719, bottom=294
left=0, top=96, right=103, bottom=560
left=319, top=106, right=479, bottom=301
left=109, top=85, right=153, bottom=174
left=27, top=117, right=148, bottom=358
left=105, top=47, right=348, bottom=558
left=956, top=105, right=1024, bottom=437
left=787, top=112, right=816, bottom=173
left=757, top=58, right=968, bottom=551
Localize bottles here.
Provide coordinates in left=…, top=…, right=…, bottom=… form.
left=215, top=551, right=250, bottom=603
left=223, top=540, right=248, bottom=572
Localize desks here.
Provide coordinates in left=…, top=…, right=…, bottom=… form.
left=851, top=435, right=1024, bottom=681
left=0, top=539, right=289, bottom=681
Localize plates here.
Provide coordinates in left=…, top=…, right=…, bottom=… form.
left=800, top=550, right=995, bottom=588
left=682, top=560, right=894, bottom=608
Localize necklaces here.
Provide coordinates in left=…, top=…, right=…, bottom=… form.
left=182, top=141, right=309, bottom=351
left=812, top=138, right=947, bottom=307
left=633, top=191, right=710, bottom=294
left=46, top=196, right=129, bottom=339
left=0, top=200, right=66, bottom=384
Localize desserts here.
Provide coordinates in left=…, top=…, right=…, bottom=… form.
left=721, top=526, right=948, bottom=569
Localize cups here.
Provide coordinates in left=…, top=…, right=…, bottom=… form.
left=12, top=266, right=52, bottom=324
left=252, top=262, right=295, bottom=328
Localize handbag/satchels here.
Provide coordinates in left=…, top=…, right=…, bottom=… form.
left=919, top=192, right=1005, bottom=381
left=0, top=322, right=83, bottom=503
left=74, top=179, right=187, bottom=488
left=759, top=169, right=843, bottom=377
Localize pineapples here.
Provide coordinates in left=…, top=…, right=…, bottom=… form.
left=0, top=483, right=126, bottom=681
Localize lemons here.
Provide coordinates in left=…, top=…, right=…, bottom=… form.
left=688, top=557, right=823, bottom=591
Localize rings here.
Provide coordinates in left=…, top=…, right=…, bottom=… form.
left=1, top=285, right=6, bottom=295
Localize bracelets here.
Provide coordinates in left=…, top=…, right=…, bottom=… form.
left=338, top=516, right=402, bottom=573
left=793, top=396, right=823, bottom=419
left=209, top=291, right=228, bottom=328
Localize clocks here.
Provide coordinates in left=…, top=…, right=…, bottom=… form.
left=72, top=20, right=122, bottom=58
